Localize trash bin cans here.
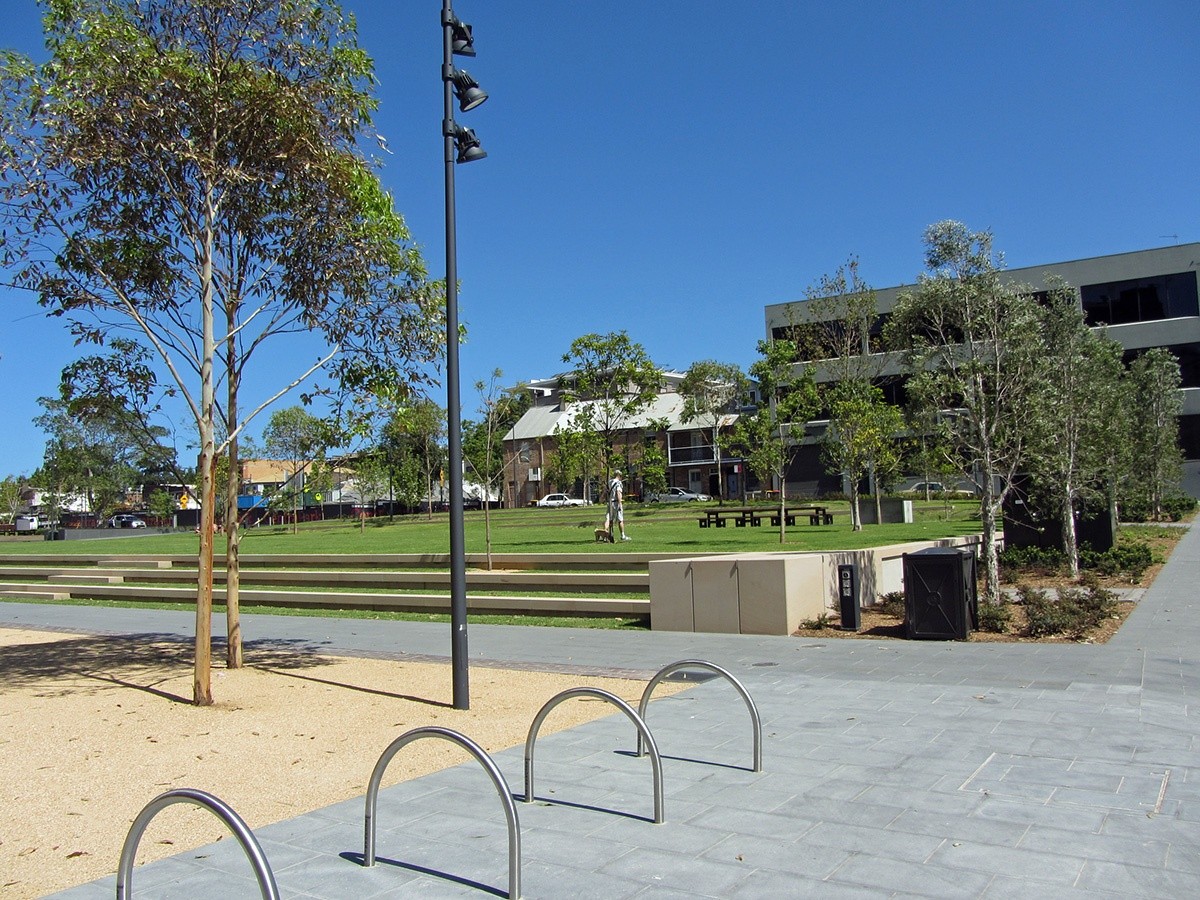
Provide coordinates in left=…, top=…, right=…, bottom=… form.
left=902, top=546, right=979, bottom=640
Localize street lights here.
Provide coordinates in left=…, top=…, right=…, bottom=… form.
left=440, top=0, right=489, bottom=711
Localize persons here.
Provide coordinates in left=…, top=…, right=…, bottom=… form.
left=604, top=469, right=631, bottom=542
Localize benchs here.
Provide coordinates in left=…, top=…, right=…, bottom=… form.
left=697, top=513, right=834, bottom=528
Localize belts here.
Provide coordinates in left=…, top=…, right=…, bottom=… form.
left=611, top=500, right=618, bottom=503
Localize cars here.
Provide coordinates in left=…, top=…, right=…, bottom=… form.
left=104, top=514, right=148, bottom=530
left=643, top=487, right=714, bottom=504
left=536, top=492, right=593, bottom=507
left=896, top=481, right=974, bottom=495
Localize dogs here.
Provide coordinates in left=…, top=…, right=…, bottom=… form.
left=594, top=528, right=615, bottom=544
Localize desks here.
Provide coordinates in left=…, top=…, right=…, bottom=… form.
left=704, top=507, right=829, bottom=526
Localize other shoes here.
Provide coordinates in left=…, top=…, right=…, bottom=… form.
left=621, top=536, right=632, bottom=542
